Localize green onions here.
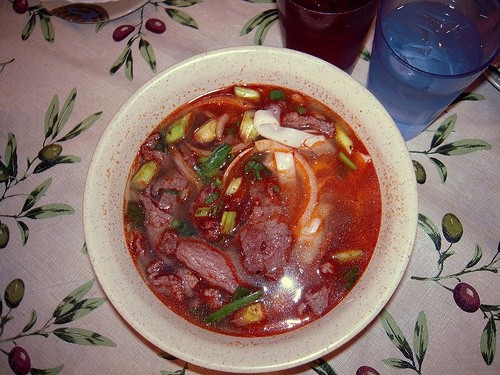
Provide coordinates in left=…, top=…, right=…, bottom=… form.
left=131, top=86, right=357, bottom=324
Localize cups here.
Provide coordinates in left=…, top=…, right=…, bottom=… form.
left=367, top=0, right=500, bottom=142
left=277, top=0, right=379, bottom=73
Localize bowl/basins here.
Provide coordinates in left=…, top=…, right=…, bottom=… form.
left=83, top=43, right=416, bottom=374
